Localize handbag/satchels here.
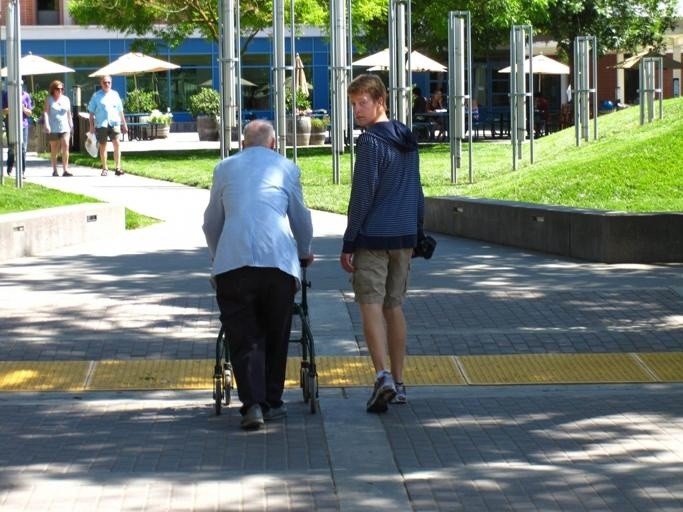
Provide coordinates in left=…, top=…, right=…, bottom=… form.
left=84, top=131, right=99, bottom=158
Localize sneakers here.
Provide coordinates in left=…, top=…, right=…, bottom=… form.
left=115, top=168, right=124, bottom=176
left=62, top=171, right=73, bottom=176
left=52, top=172, right=59, bottom=176
left=263, top=401, right=289, bottom=421
left=366, top=370, right=398, bottom=414
left=239, top=403, right=264, bottom=431
left=101, top=168, right=108, bottom=176
left=388, top=381, right=408, bottom=405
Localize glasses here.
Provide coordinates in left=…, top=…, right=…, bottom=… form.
left=54, top=86, right=64, bottom=91
left=101, top=80, right=112, bottom=85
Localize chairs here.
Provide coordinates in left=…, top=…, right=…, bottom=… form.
left=411, top=105, right=510, bottom=141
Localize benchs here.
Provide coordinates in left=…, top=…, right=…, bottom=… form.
left=125, top=122, right=148, bottom=141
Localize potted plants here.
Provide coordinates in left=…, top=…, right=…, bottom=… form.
left=188, top=88, right=220, bottom=142
left=147, top=112, right=173, bottom=137
left=281, top=84, right=311, bottom=147
left=310, top=117, right=328, bottom=147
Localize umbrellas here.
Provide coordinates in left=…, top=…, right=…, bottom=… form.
left=293, top=52, right=307, bottom=102
left=600, top=48, right=680, bottom=72
left=496, top=53, right=570, bottom=93
left=365, top=65, right=445, bottom=76
left=260, top=76, right=314, bottom=92
left=0, top=53, right=77, bottom=95
left=350, top=45, right=450, bottom=71
left=200, top=72, right=259, bottom=90
left=86, top=51, right=183, bottom=114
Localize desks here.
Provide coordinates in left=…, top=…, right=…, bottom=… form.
left=123, top=112, right=149, bottom=140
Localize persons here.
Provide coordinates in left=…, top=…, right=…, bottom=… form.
left=410, top=84, right=428, bottom=115
left=42, top=80, right=75, bottom=177
left=424, top=89, right=449, bottom=142
left=531, top=92, right=549, bottom=136
left=0, top=79, right=32, bottom=180
left=335, top=71, right=427, bottom=415
left=463, top=94, right=479, bottom=135
left=86, top=74, right=129, bottom=176
left=202, top=118, right=313, bottom=432
